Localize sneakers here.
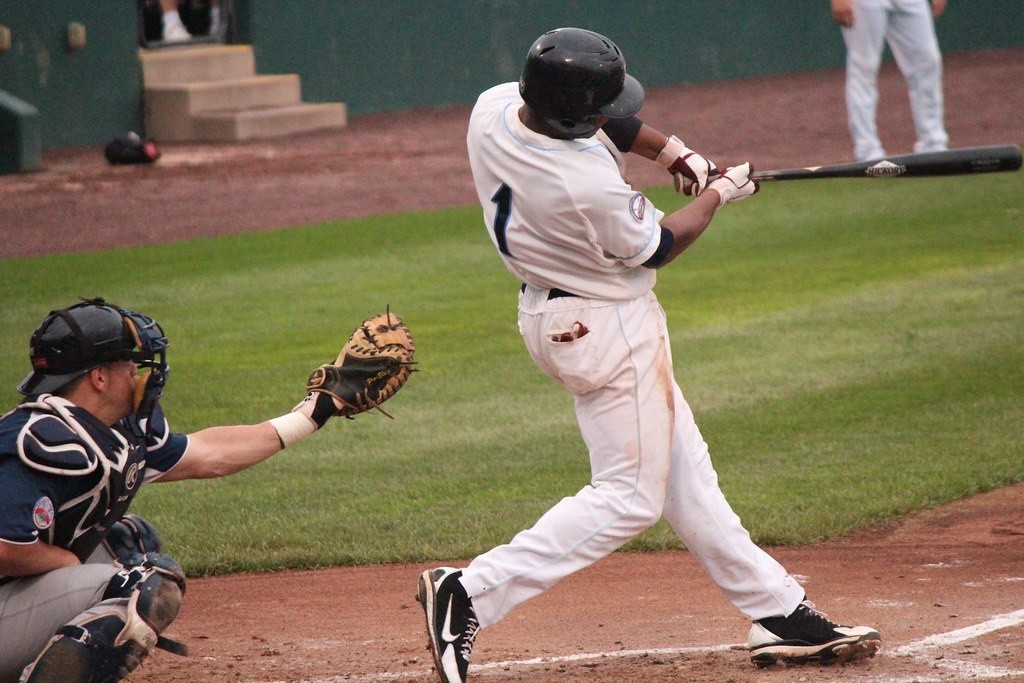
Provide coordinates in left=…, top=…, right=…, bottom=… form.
left=749, top=596, right=882, bottom=667
left=415, top=566, right=482, bottom=683
left=163, top=24, right=190, bottom=41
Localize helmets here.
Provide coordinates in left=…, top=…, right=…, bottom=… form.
left=16, top=296, right=169, bottom=418
left=518, top=27, right=645, bottom=139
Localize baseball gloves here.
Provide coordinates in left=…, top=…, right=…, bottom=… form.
left=292, top=301, right=425, bottom=431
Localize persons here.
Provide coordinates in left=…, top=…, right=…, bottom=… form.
left=419, top=27, right=881, bottom=683
left=831, top=0, right=949, bottom=162
left=1, top=296, right=415, bottom=683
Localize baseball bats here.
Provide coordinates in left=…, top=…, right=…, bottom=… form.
left=674, top=142, right=1024, bottom=196
